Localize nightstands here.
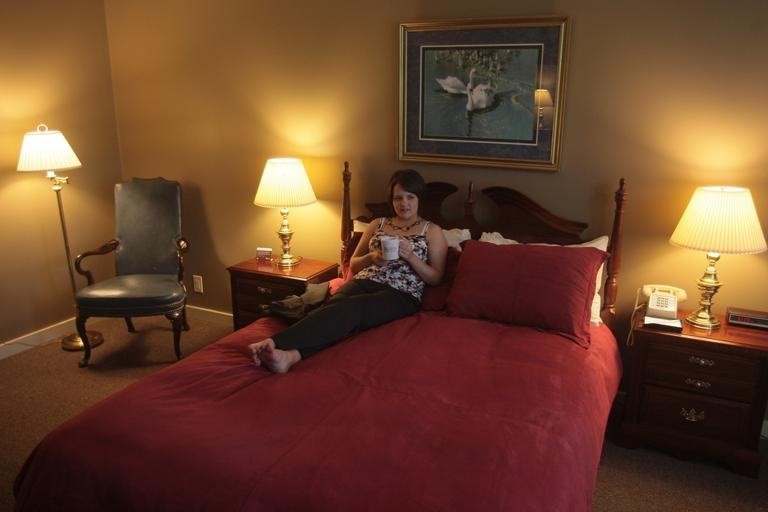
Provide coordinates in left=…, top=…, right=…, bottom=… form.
left=225, top=255, right=340, bottom=332
left=613, top=302, right=768, bottom=481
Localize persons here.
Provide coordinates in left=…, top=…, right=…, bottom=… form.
left=247, top=169, right=448, bottom=374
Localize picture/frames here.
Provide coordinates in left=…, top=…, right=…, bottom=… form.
left=396, top=11, right=573, bottom=173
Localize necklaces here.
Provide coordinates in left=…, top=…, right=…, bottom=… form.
left=386, top=216, right=422, bottom=232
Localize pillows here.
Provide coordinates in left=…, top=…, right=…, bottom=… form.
left=478, top=232, right=610, bottom=327
left=445, top=239, right=611, bottom=350
left=353, top=219, right=470, bottom=253
left=340, top=231, right=461, bottom=312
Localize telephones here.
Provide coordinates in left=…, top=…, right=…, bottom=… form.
left=641, top=283, right=687, bottom=319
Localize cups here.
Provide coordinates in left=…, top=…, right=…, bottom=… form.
left=381, top=237, right=405, bottom=261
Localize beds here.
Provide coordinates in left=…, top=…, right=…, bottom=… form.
left=10, top=161, right=629, bottom=512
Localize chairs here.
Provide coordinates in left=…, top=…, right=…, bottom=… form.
left=73, top=177, right=193, bottom=369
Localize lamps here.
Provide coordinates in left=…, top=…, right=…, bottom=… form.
left=252, top=157, right=318, bottom=267
left=667, top=185, right=767, bottom=330
left=16, top=123, right=103, bottom=352
left=534, top=89, right=554, bottom=132
